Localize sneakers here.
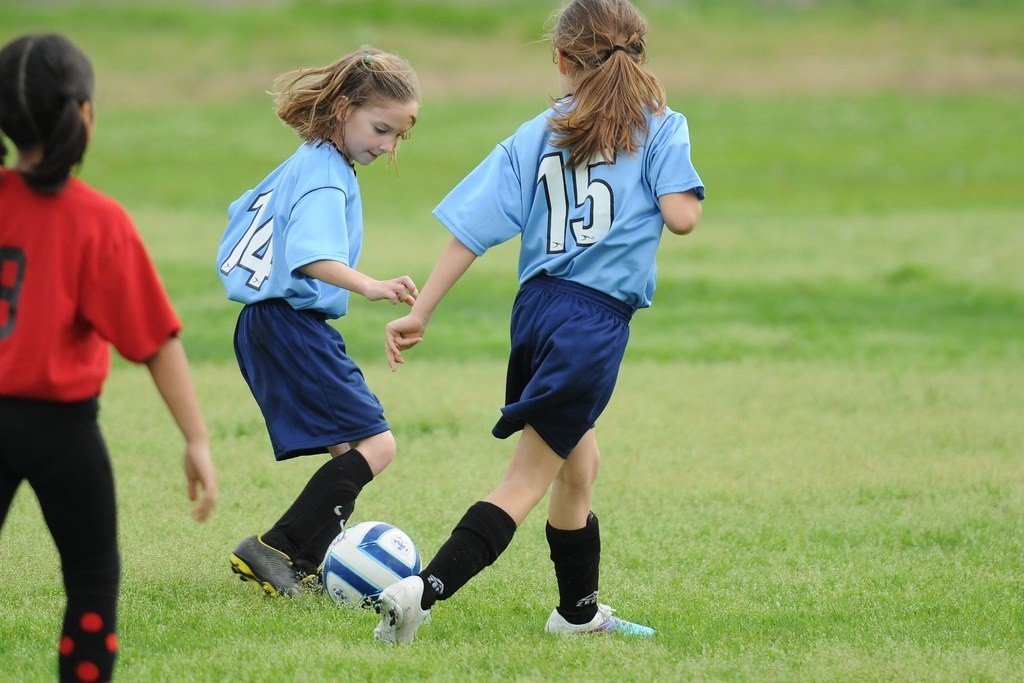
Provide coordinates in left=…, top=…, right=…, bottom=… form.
left=229, top=534, right=322, bottom=599
left=373, top=574, right=431, bottom=646
left=544, top=602, right=658, bottom=638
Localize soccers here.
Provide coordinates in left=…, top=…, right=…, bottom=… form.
left=321, top=519, right=424, bottom=615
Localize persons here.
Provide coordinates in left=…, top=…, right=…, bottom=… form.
left=216, top=48, right=421, bottom=598
left=0, top=35, right=219, bottom=683
left=374, top=0, right=705, bottom=647
